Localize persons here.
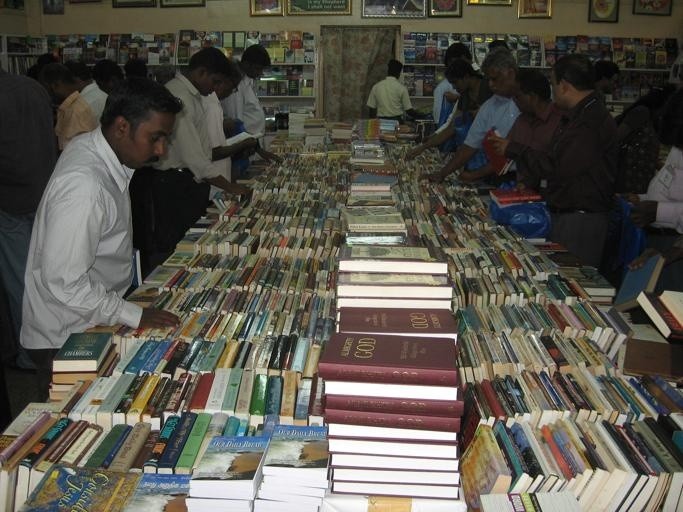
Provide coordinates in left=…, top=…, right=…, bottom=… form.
left=1, top=44, right=272, bottom=426
left=367, top=39, right=683, bottom=295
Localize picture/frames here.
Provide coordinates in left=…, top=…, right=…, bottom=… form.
left=284, top=0, right=352, bottom=16
left=2, top=0, right=208, bottom=14
left=586, top=0, right=619, bottom=23
left=466, top=0, right=512, bottom=7
left=631, top=0, right=672, bottom=16
left=516, top=0, right=551, bottom=19
left=427, top=0, right=462, bottom=18
left=360, top=0, right=428, bottom=19
left=247, top=0, right=285, bottom=17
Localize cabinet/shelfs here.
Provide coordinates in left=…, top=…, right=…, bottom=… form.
left=402, top=30, right=677, bottom=113
left=0, top=30, right=319, bottom=120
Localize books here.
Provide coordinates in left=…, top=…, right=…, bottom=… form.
left=0, top=116, right=683, bottom=512
left=1, top=33, right=177, bottom=78
left=177, top=32, right=317, bottom=131
left=404, top=32, right=543, bottom=97
left=543, top=34, right=678, bottom=116
